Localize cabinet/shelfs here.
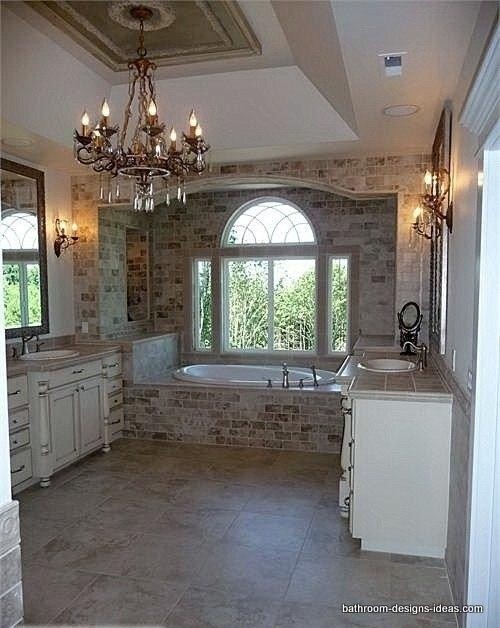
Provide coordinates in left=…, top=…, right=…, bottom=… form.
left=7, top=351, right=124, bottom=497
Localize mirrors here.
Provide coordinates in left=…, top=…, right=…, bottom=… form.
left=124, top=224, right=150, bottom=323
left=397, top=301, right=423, bottom=355
left=425, top=107, right=451, bottom=354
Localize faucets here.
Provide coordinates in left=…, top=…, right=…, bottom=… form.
left=21, top=333, right=39, bottom=354
left=402, top=340, right=427, bottom=372
left=281, top=359, right=291, bottom=388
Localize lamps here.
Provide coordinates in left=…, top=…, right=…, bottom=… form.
left=54, top=217, right=78, bottom=258
left=72, top=0, right=210, bottom=212
left=410, top=164, right=454, bottom=241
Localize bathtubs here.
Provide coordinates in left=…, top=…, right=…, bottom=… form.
left=172, top=363, right=335, bottom=387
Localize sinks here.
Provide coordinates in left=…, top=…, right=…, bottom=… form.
left=17, top=349, right=80, bottom=361
left=357, top=357, right=418, bottom=373
